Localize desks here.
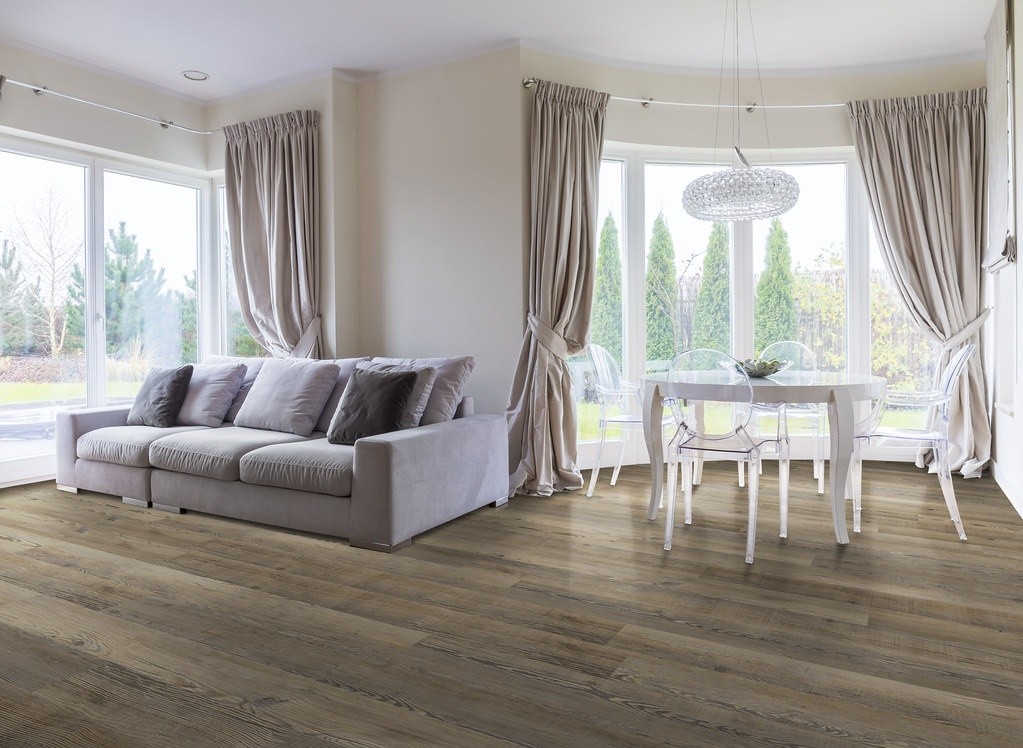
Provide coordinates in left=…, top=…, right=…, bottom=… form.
left=640, top=369, right=887, bottom=545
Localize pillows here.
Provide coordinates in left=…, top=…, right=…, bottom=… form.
left=233, top=358, right=341, bottom=436
left=199, top=356, right=265, bottom=422
left=369, top=356, right=475, bottom=425
left=292, top=356, right=370, bottom=433
left=354, top=361, right=438, bottom=430
left=175, top=363, right=247, bottom=427
left=327, top=370, right=415, bottom=446
left=126, top=364, right=193, bottom=427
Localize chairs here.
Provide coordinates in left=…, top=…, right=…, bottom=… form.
left=584, top=342, right=687, bottom=508
left=852, top=343, right=977, bottom=541
left=733, top=340, right=827, bottom=494
left=663, top=346, right=788, bottom=566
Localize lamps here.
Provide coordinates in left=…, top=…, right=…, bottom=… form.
left=682, top=0, right=801, bottom=223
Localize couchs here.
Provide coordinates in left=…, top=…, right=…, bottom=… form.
left=54, top=396, right=511, bottom=552
